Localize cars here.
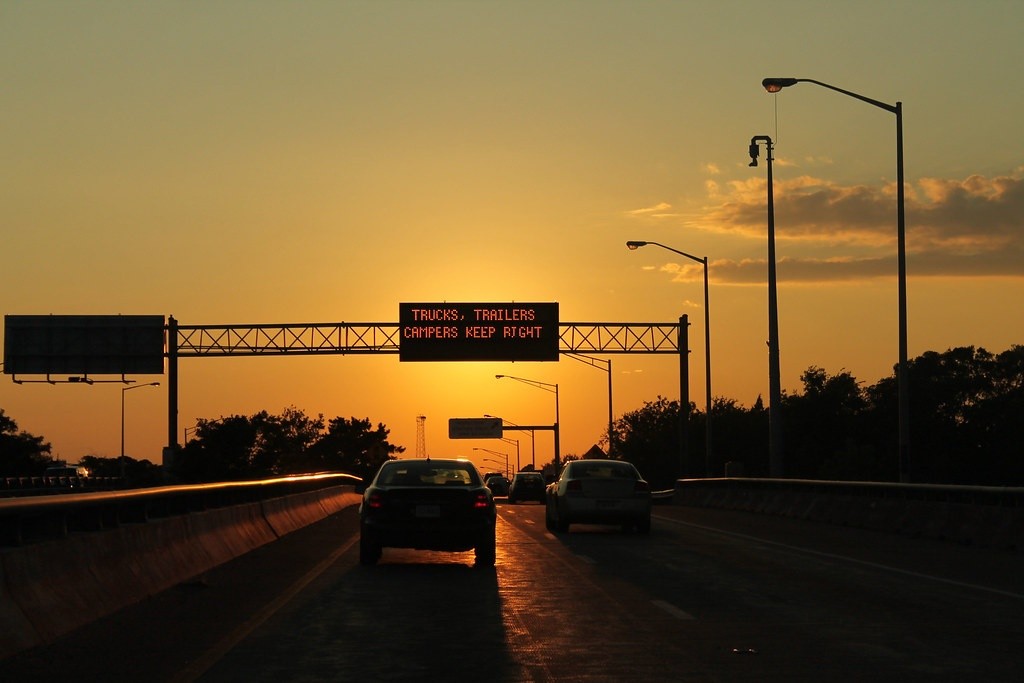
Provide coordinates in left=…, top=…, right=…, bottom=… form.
left=545, top=459, right=652, bottom=538
left=506, top=473, right=546, bottom=507
left=358, top=458, right=497, bottom=567
left=43, top=466, right=89, bottom=481
left=487, top=477, right=511, bottom=497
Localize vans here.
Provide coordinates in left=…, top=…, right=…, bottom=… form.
left=484, top=473, right=502, bottom=483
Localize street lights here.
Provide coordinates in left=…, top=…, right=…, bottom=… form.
left=762, top=77, right=914, bottom=494
left=473, top=448, right=509, bottom=479
left=485, top=414, right=535, bottom=471
left=749, top=134, right=787, bottom=484
left=122, top=381, right=160, bottom=455
left=483, top=459, right=514, bottom=480
left=495, top=374, right=559, bottom=428
left=626, top=240, right=715, bottom=474
